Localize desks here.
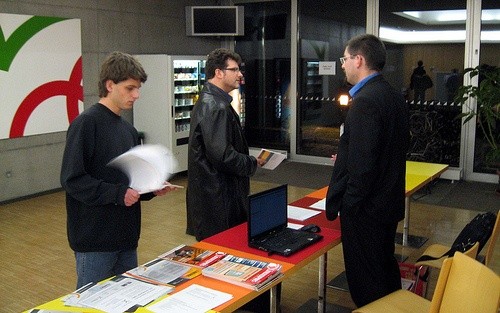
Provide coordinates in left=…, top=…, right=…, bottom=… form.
left=21, top=160, right=449, bottom=313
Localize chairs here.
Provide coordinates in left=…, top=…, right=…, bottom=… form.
left=347, top=208, right=500, bottom=313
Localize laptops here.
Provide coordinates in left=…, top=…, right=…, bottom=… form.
left=246, top=184, right=324, bottom=257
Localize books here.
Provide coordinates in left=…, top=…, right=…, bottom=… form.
left=258, top=149, right=286, bottom=170
left=202, top=254, right=285, bottom=292
left=106, top=145, right=184, bottom=194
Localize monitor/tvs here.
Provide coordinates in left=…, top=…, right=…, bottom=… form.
left=257, top=13, right=288, bottom=40
left=185, top=6, right=245, bottom=36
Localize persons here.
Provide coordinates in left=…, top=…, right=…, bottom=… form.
left=60, top=50, right=175, bottom=291
left=446, top=69, right=458, bottom=101
left=325, top=33, right=410, bottom=309
left=410, top=60, right=426, bottom=104
left=186, top=48, right=266, bottom=244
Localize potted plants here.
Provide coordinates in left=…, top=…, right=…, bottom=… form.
left=454, top=65, right=500, bottom=198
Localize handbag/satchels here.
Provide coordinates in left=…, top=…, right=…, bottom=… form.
left=448, top=211, right=497, bottom=259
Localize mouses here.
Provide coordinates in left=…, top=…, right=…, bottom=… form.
left=298, top=223, right=321, bottom=232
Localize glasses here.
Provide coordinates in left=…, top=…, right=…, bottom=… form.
left=340, top=52, right=363, bottom=65
left=217, top=67, right=240, bottom=73
